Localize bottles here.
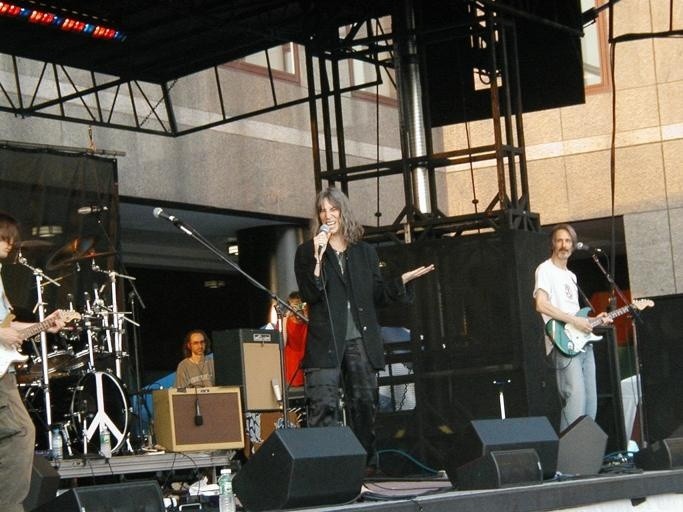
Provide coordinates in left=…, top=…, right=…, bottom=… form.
left=218, top=469, right=235, bottom=512
left=99, top=426, right=112, bottom=458
left=51, top=429, right=63, bottom=460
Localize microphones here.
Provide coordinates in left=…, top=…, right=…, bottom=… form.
left=66, top=293, right=74, bottom=302
left=152, top=207, right=179, bottom=223
left=194, top=397, right=203, bottom=427
left=83, top=291, right=90, bottom=301
left=318, top=223, right=330, bottom=255
left=77, top=205, right=109, bottom=216
left=576, top=241, right=602, bottom=253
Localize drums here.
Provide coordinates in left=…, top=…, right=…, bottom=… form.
left=17, top=313, right=107, bottom=382
left=24, top=372, right=129, bottom=459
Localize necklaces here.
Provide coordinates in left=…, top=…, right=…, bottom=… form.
left=329, top=240, right=348, bottom=255
left=190, top=357, right=205, bottom=379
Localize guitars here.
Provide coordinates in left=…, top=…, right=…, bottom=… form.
left=0, top=307, right=79, bottom=379
left=544, top=298, right=656, bottom=356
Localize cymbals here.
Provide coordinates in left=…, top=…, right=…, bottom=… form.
left=44, top=236, right=95, bottom=270
left=12, top=241, right=54, bottom=249
left=63, top=250, right=115, bottom=263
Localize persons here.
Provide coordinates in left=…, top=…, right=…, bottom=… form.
left=0, top=221, right=67, bottom=512
left=274, top=291, right=310, bottom=428
left=176, top=329, right=216, bottom=388
left=533, top=224, right=614, bottom=433
left=293, top=187, right=436, bottom=476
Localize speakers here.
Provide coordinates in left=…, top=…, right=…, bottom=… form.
left=555, top=414, right=609, bottom=474
left=443, top=415, right=559, bottom=489
left=209, top=327, right=285, bottom=412
left=416, top=228, right=566, bottom=476
left=231, top=425, right=368, bottom=512
left=29, top=479, right=166, bottom=512
left=634, top=437, right=683, bottom=471
